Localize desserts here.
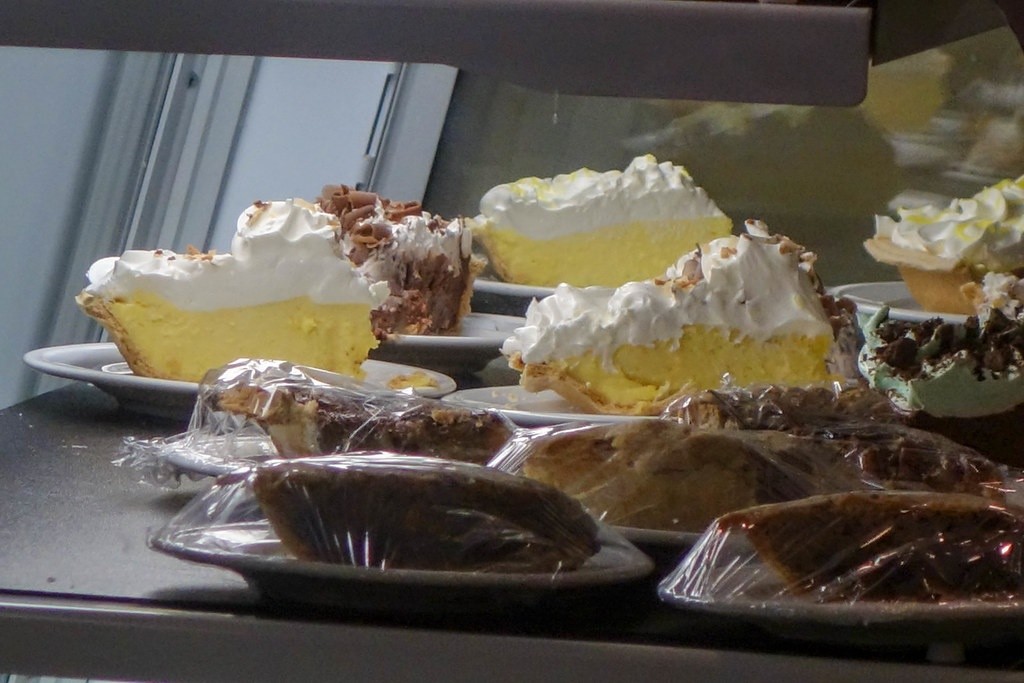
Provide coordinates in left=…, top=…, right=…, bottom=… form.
left=217, top=450, right=603, bottom=572
left=864, top=172, right=1023, bottom=315
left=498, top=219, right=861, bottom=417
left=468, top=154, right=733, bottom=289
left=715, top=490, right=1023, bottom=605
left=858, top=305, right=1024, bottom=417
left=198, top=357, right=513, bottom=466
left=518, top=384, right=1007, bottom=534
left=313, top=184, right=487, bottom=335
left=74, top=199, right=392, bottom=386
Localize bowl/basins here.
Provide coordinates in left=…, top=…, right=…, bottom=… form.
left=835, top=280, right=1022, bottom=418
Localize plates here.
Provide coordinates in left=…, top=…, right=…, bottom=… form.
left=375, top=274, right=559, bottom=371
left=19, top=343, right=681, bottom=430
left=11, top=393, right=1022, bottom=640
